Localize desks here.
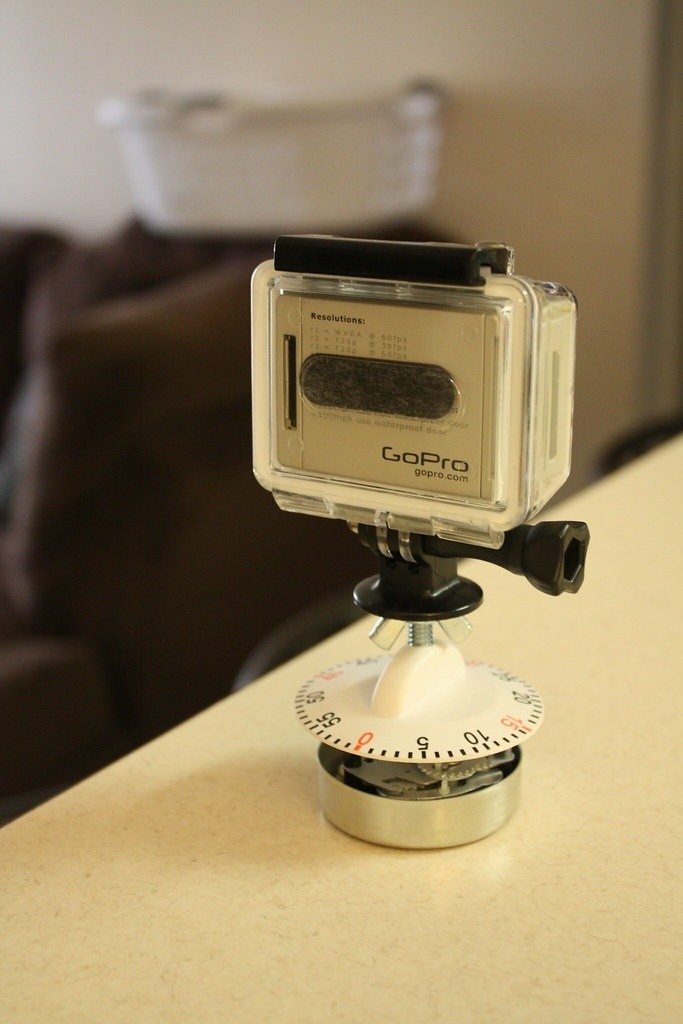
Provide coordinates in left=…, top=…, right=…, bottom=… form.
left=0, top=436, right=683, bottom=1024
